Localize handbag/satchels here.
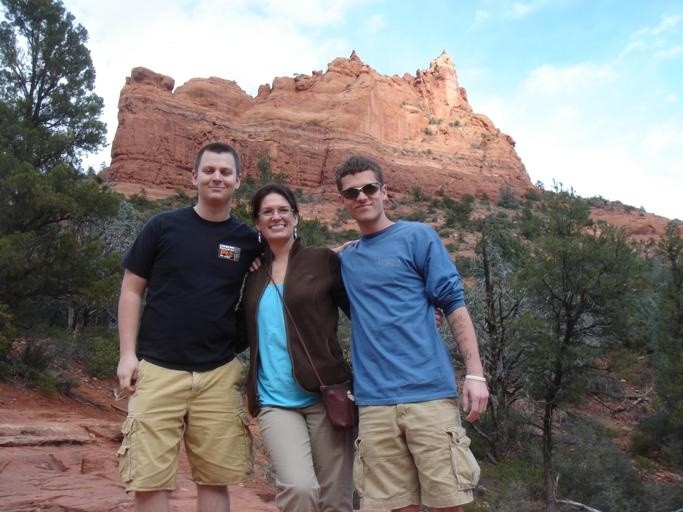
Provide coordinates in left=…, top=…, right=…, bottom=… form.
left=320, top=380, right=358, bottom=432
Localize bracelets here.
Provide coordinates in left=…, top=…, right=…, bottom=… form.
left=464, top=374, right=487, bottom=382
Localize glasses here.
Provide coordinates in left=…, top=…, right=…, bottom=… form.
left=256, top=206, right=293, bottom=215
left=342, top=182, right=381, bottom=200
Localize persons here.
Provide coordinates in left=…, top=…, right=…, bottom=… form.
left=115, top=143, right=362, bottom=511
left=246, top=184, right=443, bottom=511
left=248, top=155, right=491, bottom=511
